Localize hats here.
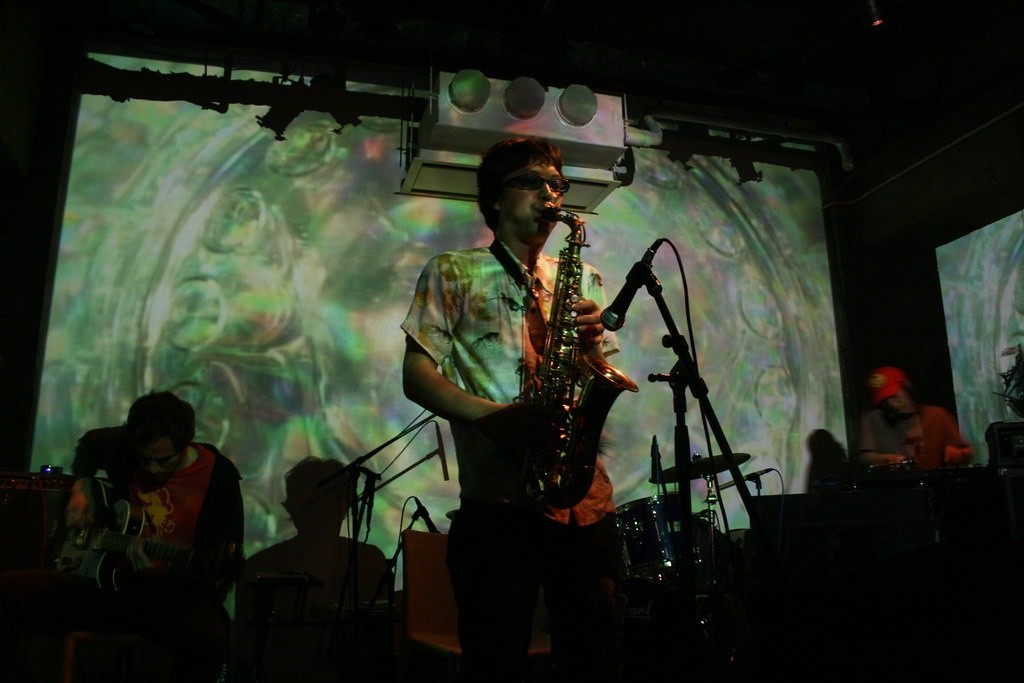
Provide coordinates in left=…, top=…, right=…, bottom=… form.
left=868, top=367, right=910, bottom=406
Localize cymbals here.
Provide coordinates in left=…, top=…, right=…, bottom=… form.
left=650, top=453, right=752, bottom=484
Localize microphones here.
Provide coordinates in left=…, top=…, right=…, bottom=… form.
left=651, top=436, right=663, bottom=482
left=435, top=423, right=449, bottom=479
left=600, top=239, right=663, bottom=330
left=414, top=496, right=440, bottom=533
left=722, top=468, right=774, bottom=490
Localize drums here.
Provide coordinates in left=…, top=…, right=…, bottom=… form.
left=612, top=490, right=702, bottom=583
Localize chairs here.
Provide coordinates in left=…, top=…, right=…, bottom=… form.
left=401, top=529, right=552, bottom=683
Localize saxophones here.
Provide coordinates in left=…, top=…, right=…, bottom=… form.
left=521, top=201, right=637, bottom=510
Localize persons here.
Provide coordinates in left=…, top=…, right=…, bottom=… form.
left=849, top=367, right=974, bottom=470
left=225, top=455, right=394, bottom=683
left=400, top=137, right=620, bottom=683
left=33, top=390, right=243, bottom=683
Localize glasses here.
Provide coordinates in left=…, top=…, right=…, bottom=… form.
left=502, top=174, right=570, bottom=193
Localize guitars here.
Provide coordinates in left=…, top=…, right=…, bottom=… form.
left=42, top=477, right=248, bottom=591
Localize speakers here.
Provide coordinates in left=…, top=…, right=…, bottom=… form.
left=0, top=471, right=86, bottom=576
